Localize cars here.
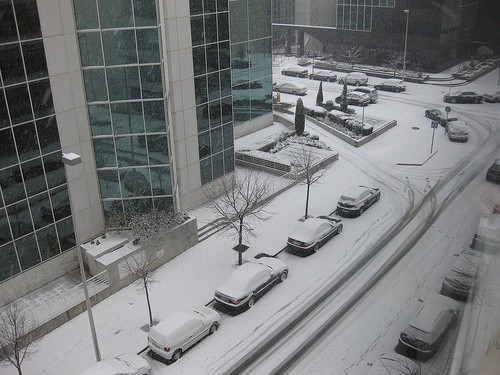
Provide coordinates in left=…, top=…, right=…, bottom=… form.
left=446, top=120, right=469, bottom=142
left=271, top=79, right=280, bottom=91
left=374, top=79, right=406, bottom=94
left=485, top=158, right=500, bottom=183
left=365, top=351, right=418, bottom=375
left=483, top=89, right=500, bottom=104
left=443, top=89, right=484, bottom=104
left=147, top=305, right=220, bottom=362
left=214, top=256, right=289, bottom=312
left=280, top=65, right=308, bottom=78
left=274, top=81, right=307, bottom=95
left=335, top=91, right=370, bottom=106
left=398, top=292, right=460, bottom=360
left=442, top=248, right=489, bottom=299
left=309, top=70, right=338, bottom=83
left=80, top=351, right=152, bottom=375
left=0, top=57, right=267, bottom=246
left=492, top=200, right=500, bottom=214
left=286, top=215, right=344, bottom=256
left=336, top=185, right=381, bottom=218
left=470, top=213, right=500, bottom=253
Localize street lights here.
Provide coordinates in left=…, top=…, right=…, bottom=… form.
left=61, top=152, right=103, bottom=363
left=402, top=9, right=410, bottom=81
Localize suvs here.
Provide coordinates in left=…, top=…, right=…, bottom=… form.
left=337, top=72, right=369, bottom=87
left=426, top=107, right=458, bottom=126
left=341, top=86, right=379, bottom=104
